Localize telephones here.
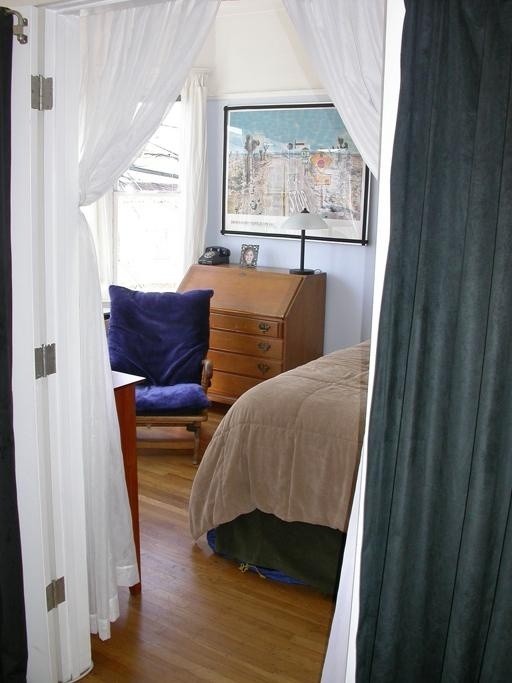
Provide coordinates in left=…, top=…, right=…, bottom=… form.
left=197, top=245, right=231, bottom=264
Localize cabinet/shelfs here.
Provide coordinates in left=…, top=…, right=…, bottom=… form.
left=178, top=264, right=328, bottom=406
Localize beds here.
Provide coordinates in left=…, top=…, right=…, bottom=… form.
left=188, top=338, right=371, bottom=602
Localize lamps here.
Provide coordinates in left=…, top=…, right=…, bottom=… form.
left=283, top=208, right=329, bottom=275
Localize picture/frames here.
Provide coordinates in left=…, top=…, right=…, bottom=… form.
left=220, top=102, right=371, bottom=247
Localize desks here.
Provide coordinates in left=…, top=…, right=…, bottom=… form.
left=111, top=368, right=148, bottom=597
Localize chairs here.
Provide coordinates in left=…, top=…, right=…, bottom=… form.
left=106, top=285, right=214, bottom=466
